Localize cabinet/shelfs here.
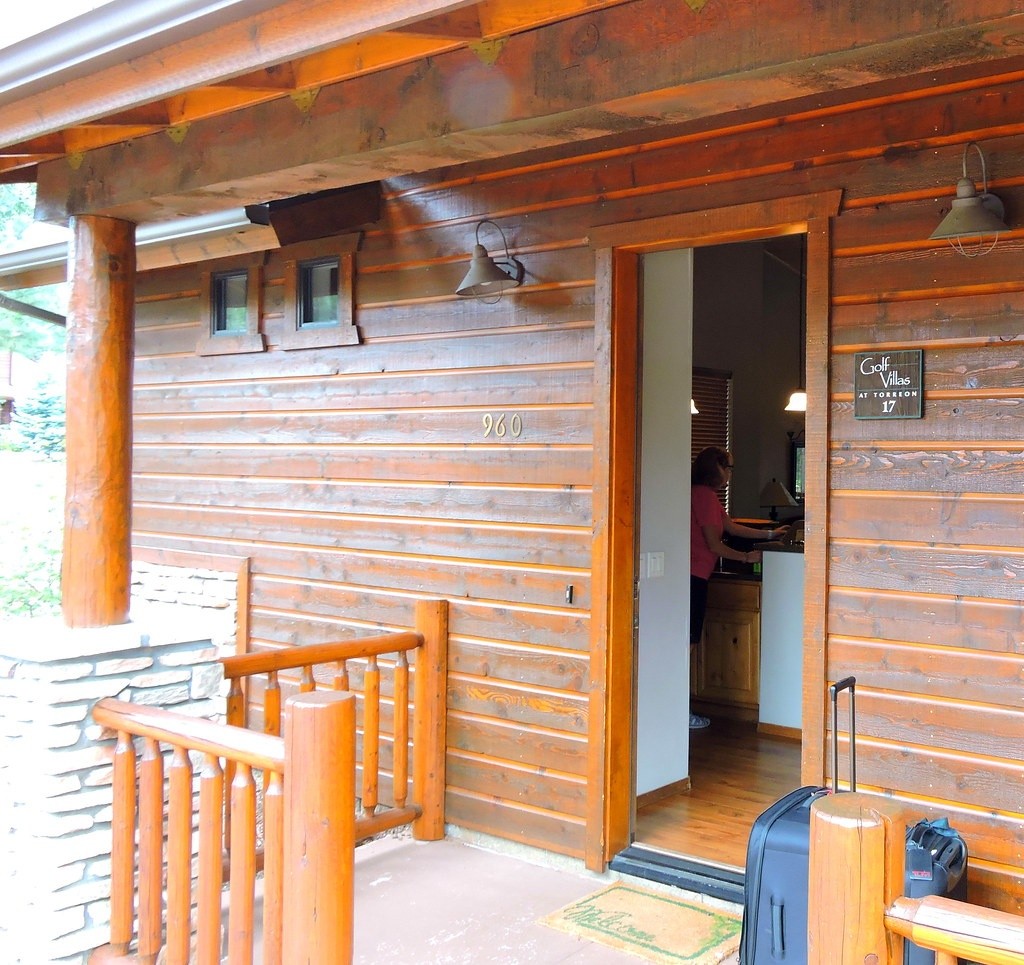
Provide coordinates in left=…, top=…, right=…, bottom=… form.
left=689, top=577, right=762, bottom=724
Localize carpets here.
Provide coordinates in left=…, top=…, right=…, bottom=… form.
left=536, top=879, right=743, bottom=965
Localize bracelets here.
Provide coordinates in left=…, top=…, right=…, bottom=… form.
left=744, top=552, right=747, bottom=563
left=768, top=529, right=772, bottom=539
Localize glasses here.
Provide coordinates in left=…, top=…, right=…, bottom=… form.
left=722, top=465, right=734, bottom=472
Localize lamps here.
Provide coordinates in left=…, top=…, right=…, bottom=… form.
left=784, top=233, right=808, bottom=412
left=455, top=219, right=524, bottom=304
left=759, top=478, right=799, bottom=521
left=928, top=141, right=1013, bottom=260
left=690, top=398, right=698, bottom=415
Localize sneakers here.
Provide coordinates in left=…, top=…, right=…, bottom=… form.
left=689, top=713, right=710, bottom=729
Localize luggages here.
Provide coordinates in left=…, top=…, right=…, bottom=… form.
left=738, top=675, right=860, bottom=964
left=891, top=819, right=969, bottom=964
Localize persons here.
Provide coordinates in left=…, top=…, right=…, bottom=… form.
left=690, top=446, right=792, bottom=730
